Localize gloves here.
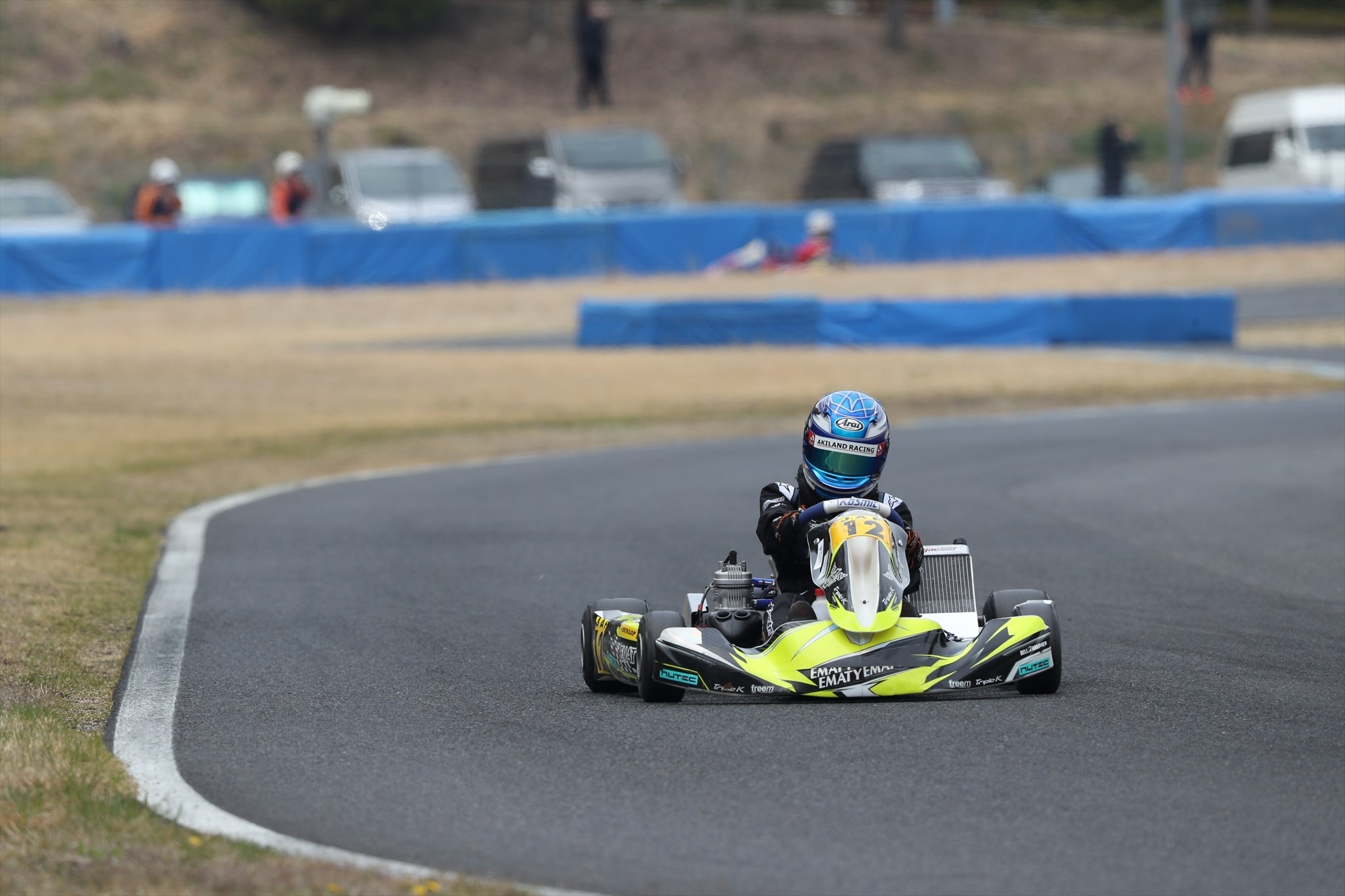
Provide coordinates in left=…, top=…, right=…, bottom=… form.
left=904, top=525, right=921, bottom=555
left=777, top=510, right=802, bottom=534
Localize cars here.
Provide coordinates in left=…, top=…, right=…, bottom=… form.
left=798, top=128, right=1018, bottom=204
left=474, top=128, right=688, bottom=211
left=303, top=146, right=478, bottom=219
left=0, top=177, right=92, bottom=236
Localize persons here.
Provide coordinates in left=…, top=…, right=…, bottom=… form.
left=1176, top=8, right=1227, bottom=89
left=795, top=207, right=836, bottom=264
left=756, top=390, right=926, bottom=624
left=1096, top=116, right=1143, bottom=198
left=134, top=156, right=180, bottom=225
left=573, top=0, right=614, bottom=108
left=267, top=147, right=311, bottom=223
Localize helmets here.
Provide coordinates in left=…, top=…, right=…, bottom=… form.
left=802, top=389, right=891, bottom=500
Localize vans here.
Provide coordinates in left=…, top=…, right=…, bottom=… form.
left=1212, top=80, right=1345, bottom=195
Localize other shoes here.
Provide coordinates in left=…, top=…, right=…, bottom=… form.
left=787, top=601, right=817, bottom=622
left=900, top=599, right=919, bottom=617
